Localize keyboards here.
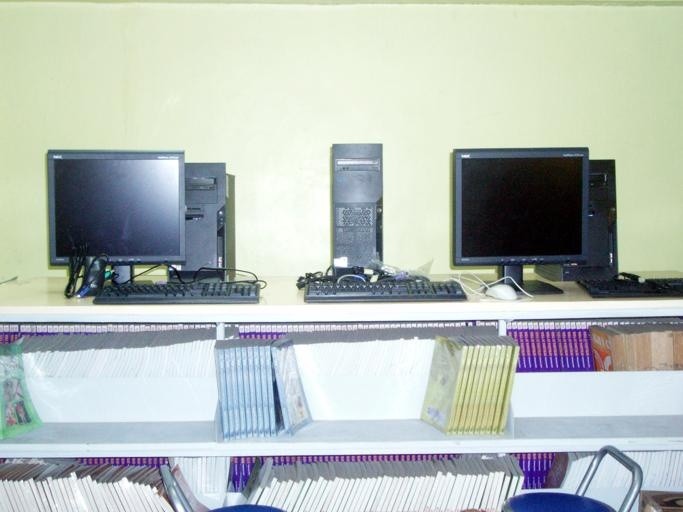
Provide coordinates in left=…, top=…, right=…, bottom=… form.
left=580, top=278, right=683, bottom=299
left=93, top=283, right=261, bottom=305
left=303, top=279, right=466, bottom=303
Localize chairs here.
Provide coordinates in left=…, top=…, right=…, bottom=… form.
left=158, top=464, right=287, bottom=512
left=501, top=445, right=643, bottom=512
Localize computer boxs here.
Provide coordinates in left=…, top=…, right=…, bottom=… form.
left=535, top=158, right=618, bottom=282
left=168, top=162, right=226, bottom=283
left=330, top=143, right=384, bottom=276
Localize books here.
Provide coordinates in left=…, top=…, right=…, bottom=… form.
left=214, top=320, right=520, bottom=440
left=2, top=323, right=216, bottom=440
left=1, top=450, right=683, bottom=512
left=506, top=319, right=682, bottom=372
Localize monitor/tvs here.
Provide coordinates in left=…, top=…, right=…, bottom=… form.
left=45, top=148, right=187, bottom=281
left=450, top=145, right=589, bottom=297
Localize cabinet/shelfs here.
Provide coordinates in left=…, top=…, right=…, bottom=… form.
left=0, top=270, right=683, bottom=458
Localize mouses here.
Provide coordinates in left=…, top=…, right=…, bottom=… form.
left=484, top=282, right=518, bottom=300
